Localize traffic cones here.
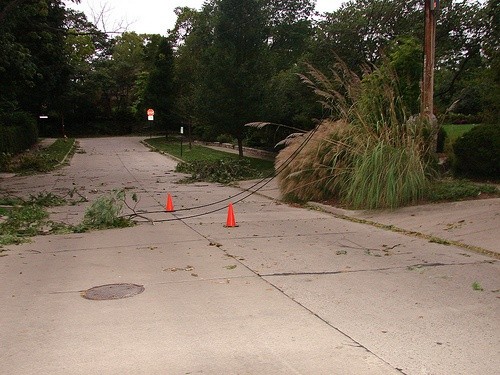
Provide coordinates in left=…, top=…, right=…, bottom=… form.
left=223, top=203, right=239, bottom=228
left=165, top=194, right=176, bottom=212
